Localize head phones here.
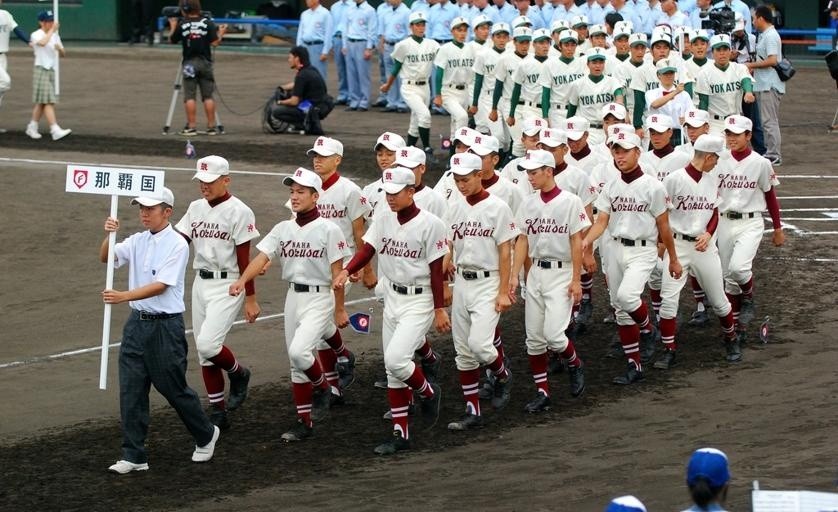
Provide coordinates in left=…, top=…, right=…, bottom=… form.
left=182, top=0, right=192, bottom=13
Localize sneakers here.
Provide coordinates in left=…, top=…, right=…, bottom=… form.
left=281, top=416, right=312, bottom=443
left=333, top=100, right=407, bottom=112
left=191, top=367, right=250, bottom=462
left=374, top=351, right=441, bottom=455
left=309, top=351, right=356, bottom=423
left=108, top=460, right=149, bottom=474
left=176, top=126, right=197, bottom=136
left=199, top=128, right=216, bottom=135
left=50, top=126, right=72, bottom=140
left=424, top=147, right=439, bottom=164
left=25, top=124, right=42, bottom=139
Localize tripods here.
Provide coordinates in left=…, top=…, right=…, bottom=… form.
left=162, top=56, right=225, bottom=135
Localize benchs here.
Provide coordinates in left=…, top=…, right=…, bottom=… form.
left=156, top=18, right=298, bottom=48
left=755, top=29, right=838, bottom=55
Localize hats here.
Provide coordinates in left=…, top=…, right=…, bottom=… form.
left=130, top=186, right=174, bottom=208
left=38, top=10, right=54, bottom=21
left=686, top=448, right=730, bottom=487
left=282, top=131, right=425, bottom=194
left=605, top=495, right=647, bottom=512
left=656, top=57, right=677, bottom=72
left=823, top=0, right=838, bottom=12
left=192, top=155, right=230, bottom=183
left=588, top=46, right=606, bottom=60
left=732, top=11, right=745, bottom=32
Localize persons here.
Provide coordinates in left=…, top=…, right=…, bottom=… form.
left=171, top=153, right=261, bottom=432
left=232, top=0, right=838, bottom=454
left=25, top=9, right=72, bottom=141
left=99, top=188, right=221, bottom=475
left=0, top=9, right=33, bottom=133
left=680, top=447, right=732, bottom=511
left=168, top=1, right=229, bottom=137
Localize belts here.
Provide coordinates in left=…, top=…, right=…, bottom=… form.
left=449, top=84, right=464, bottom=89
left=721, top=211, right=761, bottom=219
left=458, top=233, right=698, bottom=281
left=517, top=101, right=542, bottom=108
left=712, top=115, right=730, bottom=120
left=557, top=105, right=568, bottom=109
left=435, top=40, right=452, bottom=43
left=408, top=81, right=425, bottom=85
left=303, top=35, right=396, bottom=46
left=200, top=269, right=422, bottom=295
left=132, top=310, right=182, bottom=320
left=589, top=124, right=604, bottom=128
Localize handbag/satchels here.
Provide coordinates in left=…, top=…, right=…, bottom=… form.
left=774, top=57, right=796, bottom=81
